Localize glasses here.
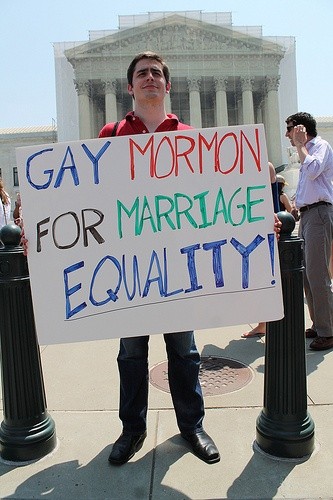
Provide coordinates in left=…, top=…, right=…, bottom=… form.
left=287, top=126, right=294, bottom=132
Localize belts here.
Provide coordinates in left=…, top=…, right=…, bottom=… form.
left=300, top=201, right=332, bottom=212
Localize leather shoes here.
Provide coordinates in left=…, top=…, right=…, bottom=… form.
left=310, top=336, right=333, bottom=350
left=181, top=428, right=220, bottom=464
left=109, top=428, right=148, bottom=465
left=306, top=326, right=317, bottom=338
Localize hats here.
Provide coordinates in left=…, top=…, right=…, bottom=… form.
left=277, top=175, right=289, bottom=186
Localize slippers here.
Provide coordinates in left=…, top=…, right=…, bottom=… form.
left=241, top=330, right=266, bottom=337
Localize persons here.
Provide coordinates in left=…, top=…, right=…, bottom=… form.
left=19, top=51, right=282, bottom=466
left=241, top=162, right=294, bottom=337
left=13, top=194, right=22, bottom=218
left=285, top=112, right=333, bottom=348
left=0, top=176, right=11, bottom=231
